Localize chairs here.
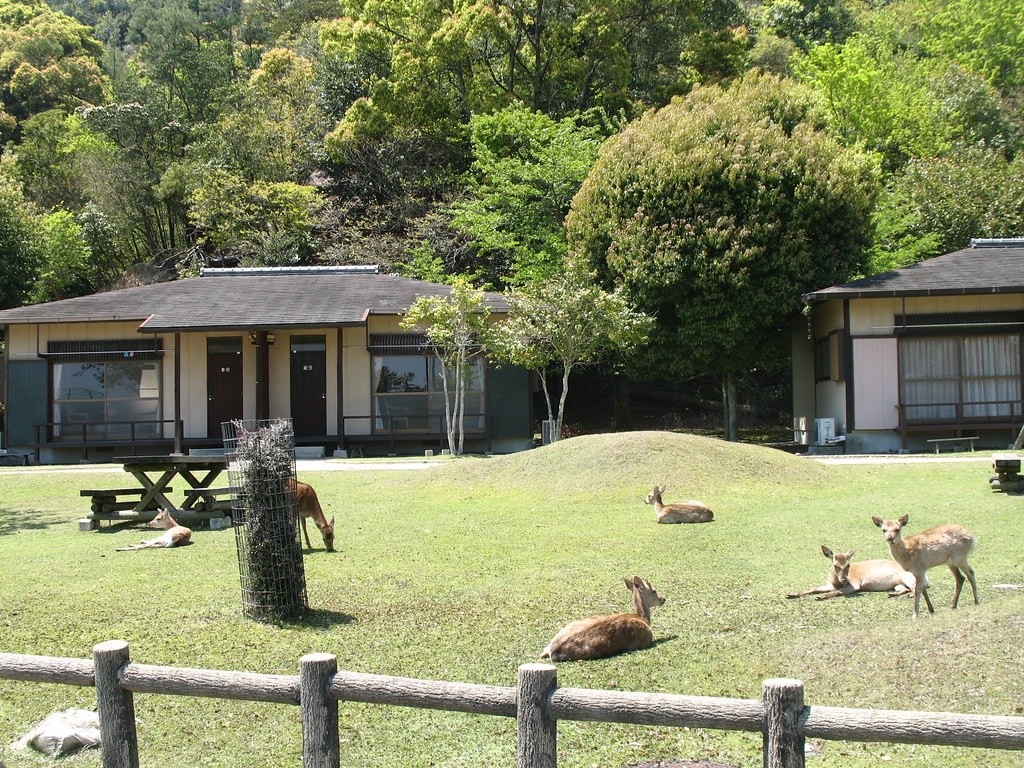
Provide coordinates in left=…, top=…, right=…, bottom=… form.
left=65, top=403, right=95, bottom=435
left=383, top=398, right=410, bottom=428
left=127, top=402, right=157, bottom=433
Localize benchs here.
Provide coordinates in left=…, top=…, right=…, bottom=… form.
left=81, top=486, right=173, bottom=512
left=185, top=486, right=241, bottom=510
left=991, top=452, right=1022, bottom=483
left=927, top=437, right=979, bottom=454
left=0, top=453, right=34, bottom=466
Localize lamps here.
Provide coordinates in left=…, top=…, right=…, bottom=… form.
left=267, top=334, right=276, bottom=344
left=249, top=333, right=257, bottom=345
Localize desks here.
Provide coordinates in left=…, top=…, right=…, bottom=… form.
left=103, top=408, right=119, bottom=434
left=87, top=455, right=226, bottom=518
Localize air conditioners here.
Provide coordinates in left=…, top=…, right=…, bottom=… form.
left=814, top=417, right=835, bottom=446
left=542, top=420, right=556, bottom=444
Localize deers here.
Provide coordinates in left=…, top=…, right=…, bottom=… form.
left=785, top=545, right=929, bottom=601
left=116, top=508, right=191, bottom=552
left=871, top=514, right=980, bottom=617
left=288, top=479, right=335, bottom=552
left=644, top=484, right=714, bottom=524
left=538, top=576, right=666, bottom=661
